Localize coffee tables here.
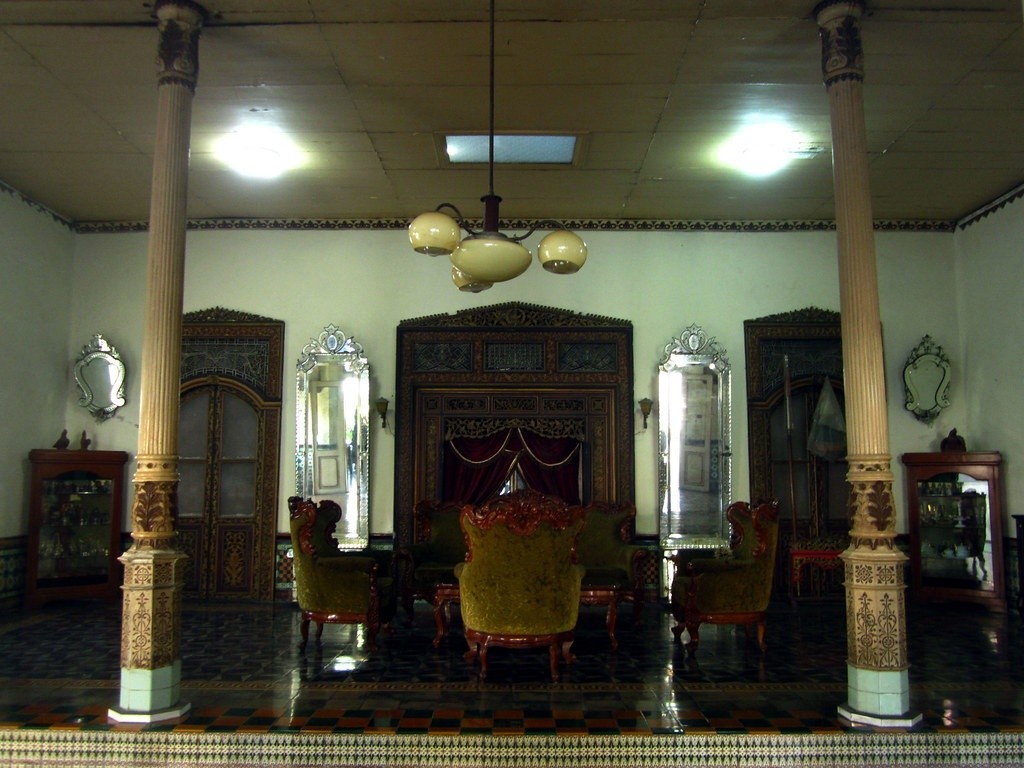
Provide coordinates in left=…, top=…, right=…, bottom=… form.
left=430, top=572, right=637, bottom=654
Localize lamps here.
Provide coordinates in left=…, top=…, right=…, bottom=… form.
left=408, top=0, right=589, bottom=293
left=638, top=396, right=653, bottom=428
left=376, top=396, right=389, bottom=430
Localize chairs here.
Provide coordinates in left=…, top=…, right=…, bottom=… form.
left=671, top=496, right=783, bottom=658
left=403, top=498, right=467, bottom=637
left=288, top=496, right=398, bottom=653
left=453, top=487, right=587, bottom=683
left=572, top=501, right=639, bottom=650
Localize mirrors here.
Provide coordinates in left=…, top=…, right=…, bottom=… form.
left=657, top=324, right=733, bottom=549
left=296, top=323, right=372, bottom=553
left=73, top=333, right=128, bottom=422
left=901, top=334, right=953, bottom=428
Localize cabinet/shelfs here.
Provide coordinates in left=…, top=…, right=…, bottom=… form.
left=178, top=307, right=285, bottom=615
left=27, top=448, right=130, bottom=610
left=744, top=306, right=851, bottom=612
left=899, top=450, right=1008, bottom=613
